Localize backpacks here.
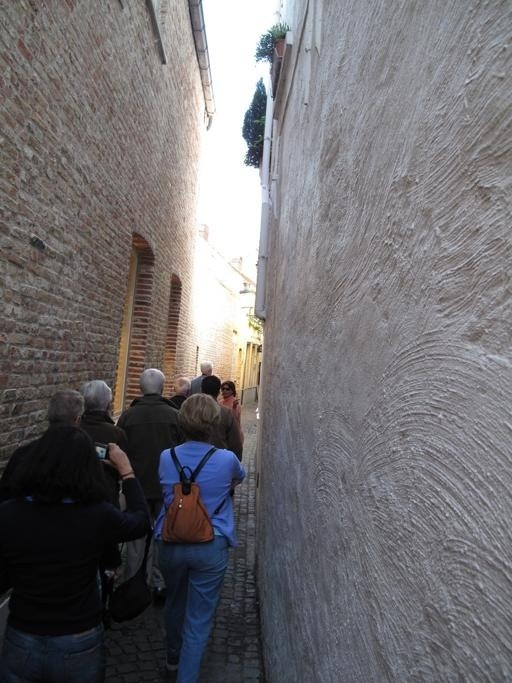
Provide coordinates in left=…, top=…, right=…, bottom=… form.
left=161, top=445, right=220, bottom=544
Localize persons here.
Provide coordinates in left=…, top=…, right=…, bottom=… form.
left=200, top=375, right=243, bottom=463
left=0, top=389, right=86, bottom=498
left=0, top=426, right=153, bottom=683
left=218, top=380, right=245, bottom=444
left=78, top=381, right=129, bottom=629
left=188, top=361, right=213, bottom=395
left=168, top=377, right=192, bottom=409
left=153, top=392, right=246, bottom=683
left=116, top=369, right=186, bottom=599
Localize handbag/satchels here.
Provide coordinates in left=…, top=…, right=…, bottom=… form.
left=108, top=570, right=153, bottom=622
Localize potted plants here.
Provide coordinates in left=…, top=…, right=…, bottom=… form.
left=254, top=19, right=291, bottom=100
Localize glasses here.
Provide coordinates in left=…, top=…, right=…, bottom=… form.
left=221, top=386, right=230, bottom=391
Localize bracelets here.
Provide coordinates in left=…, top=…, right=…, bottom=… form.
left=120, top=470, right=134, bottom=478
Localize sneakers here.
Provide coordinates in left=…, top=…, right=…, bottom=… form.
left=165, top=649, right=180, bottom=672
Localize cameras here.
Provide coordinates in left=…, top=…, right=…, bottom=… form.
left=93, top=443, right=109, bottom=463
